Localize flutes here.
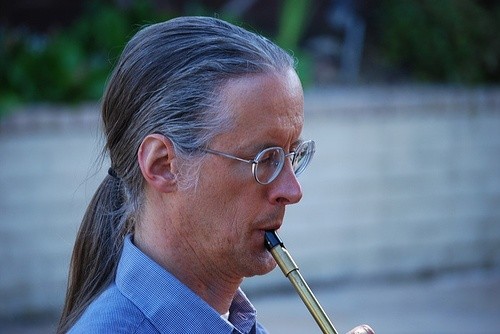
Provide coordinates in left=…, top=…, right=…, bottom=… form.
left=264, top=229, right=338, bottom=334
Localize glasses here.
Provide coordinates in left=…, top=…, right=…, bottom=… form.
left=196, top=139, right=317, bottom=186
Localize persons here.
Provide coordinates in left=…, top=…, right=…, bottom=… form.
left=52, top=17, right=375, bottom=333
